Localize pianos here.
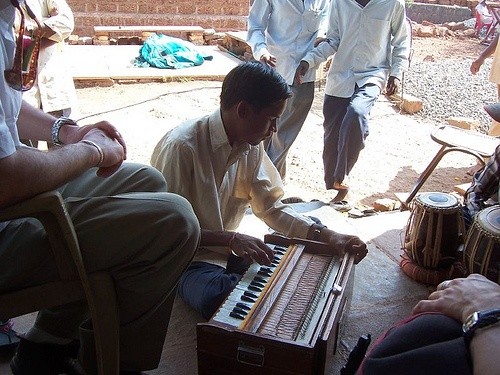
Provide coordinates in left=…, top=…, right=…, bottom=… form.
left=196, top=234, right=355, bottom=375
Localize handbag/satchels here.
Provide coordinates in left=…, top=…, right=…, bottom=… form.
left=4, top=0, right=41, bottom=92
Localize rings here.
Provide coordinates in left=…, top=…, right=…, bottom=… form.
left=441, top=280, right=452, bottom=289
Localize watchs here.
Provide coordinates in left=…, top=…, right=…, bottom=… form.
left=51, top=116, right=78, bottom=148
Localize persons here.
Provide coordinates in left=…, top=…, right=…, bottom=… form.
left=246, top=0, right=331, bottom=180
left=0, top=0, right=200, bottom=375
left=470, top=25, right=500, bottom=101
left=474, top=0, right=493, bottom=39
left=294, top=0, right=410, bottom=190
left=149, top=61, right=368, bottom=320
left=13, top=0, right=75, bottom=153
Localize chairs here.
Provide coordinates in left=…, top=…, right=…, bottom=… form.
left=406, top=124, right=500, bottom=204
left=0, top=190, right=120, bottom=375
left=475, top=10, right=495, bottom=41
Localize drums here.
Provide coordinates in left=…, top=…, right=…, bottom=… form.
left=463, top=204, right=500, bottom=286
left=403, top=191, right=460, bottom=271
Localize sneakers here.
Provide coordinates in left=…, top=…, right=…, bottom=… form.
left=9, top=333, right=84, bottom=375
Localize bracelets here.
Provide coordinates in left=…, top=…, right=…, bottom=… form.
left=228, top=231, right=239, bottom=247
left=76, top=140, right=104, bottom=167
left=462, top=308, right=500, bottom=350
left=312, top=225, right=327, bottom=241
